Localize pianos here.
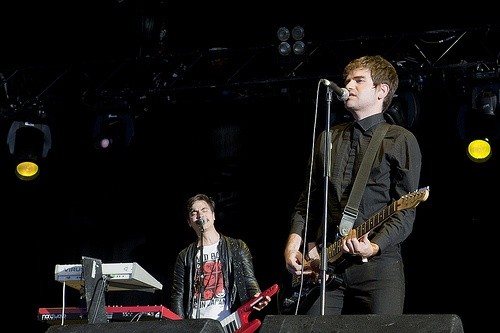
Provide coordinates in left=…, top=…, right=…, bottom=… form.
left=38, top=262, right=183, bottom=322
left=216, top=281, right=279, bottom=333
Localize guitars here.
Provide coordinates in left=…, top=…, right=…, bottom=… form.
left=276, top=185, right=431, bottom=315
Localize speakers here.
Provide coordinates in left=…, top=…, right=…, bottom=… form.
left=47, top=317, right=226, bottom=333
left=261, top=315, right=463, bottom=333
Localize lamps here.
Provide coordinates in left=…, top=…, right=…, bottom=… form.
left=82, top=0, right=500, bottom=163
left=0, top=96, right=52, bottom=181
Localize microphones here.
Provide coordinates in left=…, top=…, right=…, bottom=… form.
left=195, top=217, right=209, bottom=225
left=322, top=79, right=350, bottom=101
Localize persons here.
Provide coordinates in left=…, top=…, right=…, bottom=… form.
left=170, top=195, right=271, bottom=333
left=286, top=55, right=420, bottom=316
